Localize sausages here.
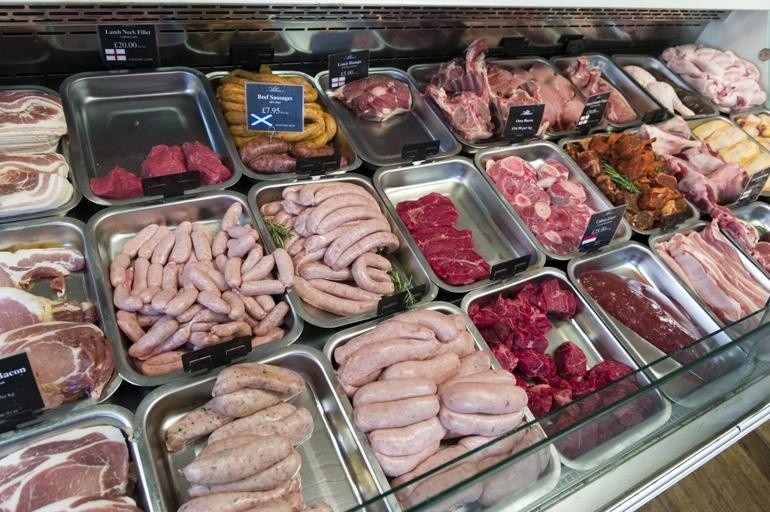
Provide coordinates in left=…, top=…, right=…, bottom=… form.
left=217, top=68, right=339, bottom=173
left=332, top=309, right=552, bottom=512
left=108, top=201, right=294, bottom=377
left=259, top=181, right=401, bottom=318
left=164, top=362, right=333, bottom=512
